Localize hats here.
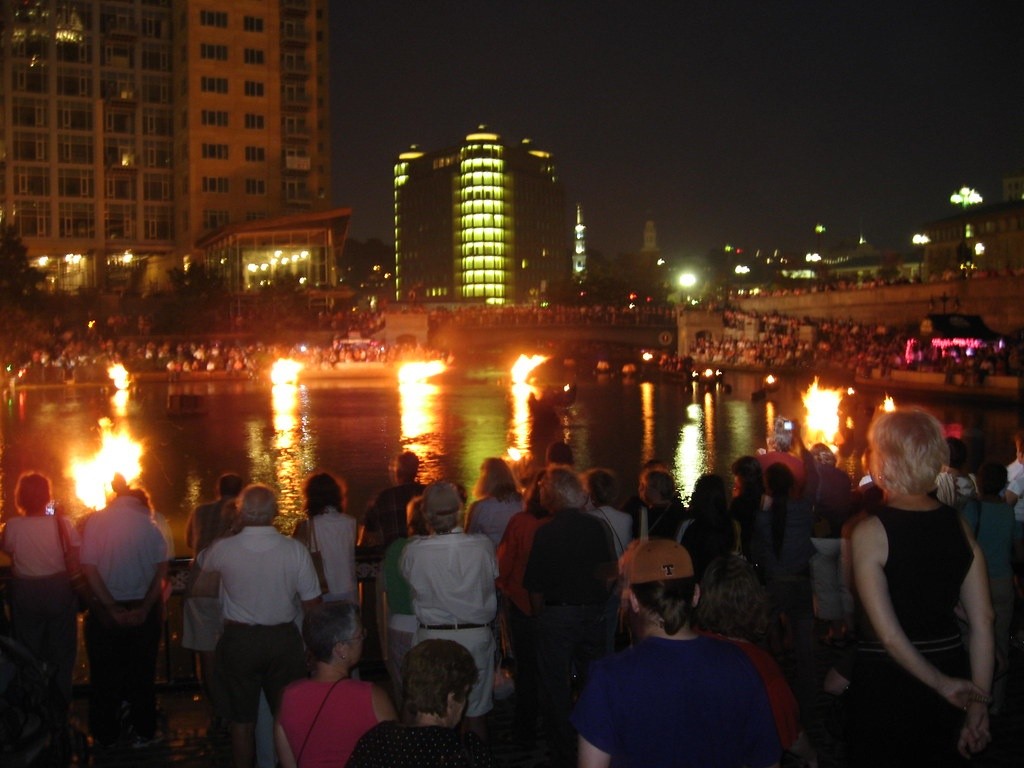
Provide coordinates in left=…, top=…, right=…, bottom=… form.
left=617, top=536, right=694, bottom=584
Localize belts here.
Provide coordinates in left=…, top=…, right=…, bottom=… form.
left=851, top=634, right=962, bottom=653
left=419, top=623, right=489, bottom=630
left=543, top=600, right=606, bottom=608
left=224, top=623, right=298, bottom=633
left=115, top=600, right=143, bottom=609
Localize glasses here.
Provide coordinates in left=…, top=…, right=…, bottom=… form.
left=333, top=627, right=367, bottom=647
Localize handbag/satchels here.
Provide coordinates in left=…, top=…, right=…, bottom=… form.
left=308, top=516, right=329, bottom=595
left=57, top=514, right=90, bottom=613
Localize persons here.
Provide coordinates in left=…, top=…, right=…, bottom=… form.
left=0, top=265, right=1024, bottom=768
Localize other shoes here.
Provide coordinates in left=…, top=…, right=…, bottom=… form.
left=823, top=635, right=845, bottom=647
left=128, top=726, right=165, bottom=749
left=831, top=630, right=864, bottom=649
left=86, top=720, right=120, bottom=749
left=782, top=648, right=799, bottom=670
left=496, top=730, right=537, bottom=748
left=206, top=717, right=229, bottom=739
left=988, top=702, right=1008, bottom=715
left=494, top=677, right=516, bottom=702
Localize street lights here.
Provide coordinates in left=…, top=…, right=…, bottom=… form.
left=912, top=233, right=930, bottom=283
left=805, top=252, right=821, bottom=294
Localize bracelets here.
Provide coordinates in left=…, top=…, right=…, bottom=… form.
left=969, top=695, right=994, bottom=705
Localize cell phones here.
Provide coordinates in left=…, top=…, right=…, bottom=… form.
left=783, top=421, right=794, bottom=430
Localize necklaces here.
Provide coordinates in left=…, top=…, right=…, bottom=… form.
left=644, top=628, right=694, bottom=636
